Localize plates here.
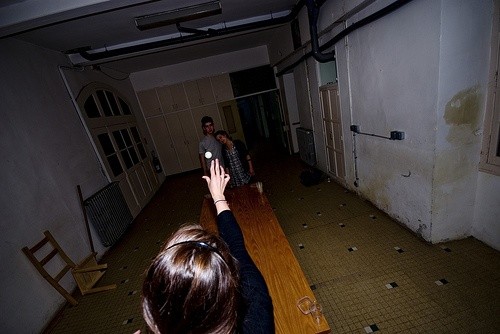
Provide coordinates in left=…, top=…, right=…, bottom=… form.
left=296, top=296, right=315, bottom=315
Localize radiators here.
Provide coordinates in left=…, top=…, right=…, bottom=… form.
left=82, top=181, right=134, bottom=248
left=295, top=126, right=317, bottom=168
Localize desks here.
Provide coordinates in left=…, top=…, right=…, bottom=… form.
left=197, top=183, right=331, bottom=334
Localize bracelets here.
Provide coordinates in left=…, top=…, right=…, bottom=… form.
left=215, top=200, right=226, bottom=204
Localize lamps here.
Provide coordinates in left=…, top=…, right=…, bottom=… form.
left=134, top=0, right=223, bottom=31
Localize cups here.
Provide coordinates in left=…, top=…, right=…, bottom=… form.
left=256, top=182, right=263, bottom=192
left=310, top=302, right=323, bottom=324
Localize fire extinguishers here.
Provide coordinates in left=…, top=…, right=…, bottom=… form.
left=152, top=157, right=162, bottom=174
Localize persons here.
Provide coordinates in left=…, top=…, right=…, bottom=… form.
left=133, top=159, right=275, bottom=334
left=199, top=116, right=224, bottom=177
left=214, top=130, right=255, bottom=188
